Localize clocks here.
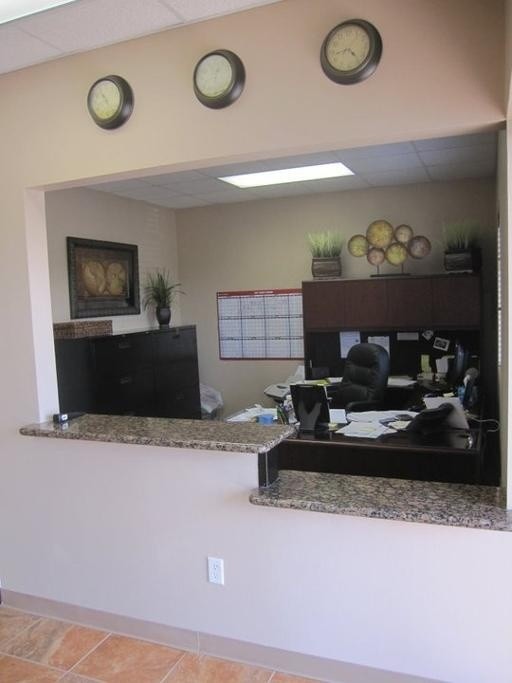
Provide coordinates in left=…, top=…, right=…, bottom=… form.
left=316, top=18, right=387, bottom=85
left=86, top=76, right=132, bottom=128
left=191, top=48, right=247, bottom=106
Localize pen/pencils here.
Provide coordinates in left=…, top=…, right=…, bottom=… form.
left=275, top=402, right=289, bottom=424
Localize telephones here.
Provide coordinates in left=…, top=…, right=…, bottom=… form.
left=405, top=402, right=455, bottom=435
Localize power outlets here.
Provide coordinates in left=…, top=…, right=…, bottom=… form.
left=207, top=555, right=223, bottom=583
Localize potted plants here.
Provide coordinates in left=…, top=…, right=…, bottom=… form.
left=142, top=267, right=189, bottom=329
left=307, top=230, right=346, bottom=280
left=442, top=220, right=484, bottom=273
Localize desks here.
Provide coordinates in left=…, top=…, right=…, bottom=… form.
left=337, top=383, right=416, bottom=391
left=280, top=411, right=496, bottom=484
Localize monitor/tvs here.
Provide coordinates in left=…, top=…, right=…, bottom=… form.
left=288, top=381, right=332, bottom=432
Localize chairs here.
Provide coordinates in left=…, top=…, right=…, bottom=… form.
left=314, top=340, right=392, bottom=416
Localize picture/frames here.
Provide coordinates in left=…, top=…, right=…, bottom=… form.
left=65, top=236, right=141, bottom=318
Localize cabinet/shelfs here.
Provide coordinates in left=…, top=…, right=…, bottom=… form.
left=388, top=275, right=484, bottom=332
left=302, top=279, right=387, bottom=332
left=54, top=329, right=153, bottom=416
left=153, top=324, right=202, bottom=419
